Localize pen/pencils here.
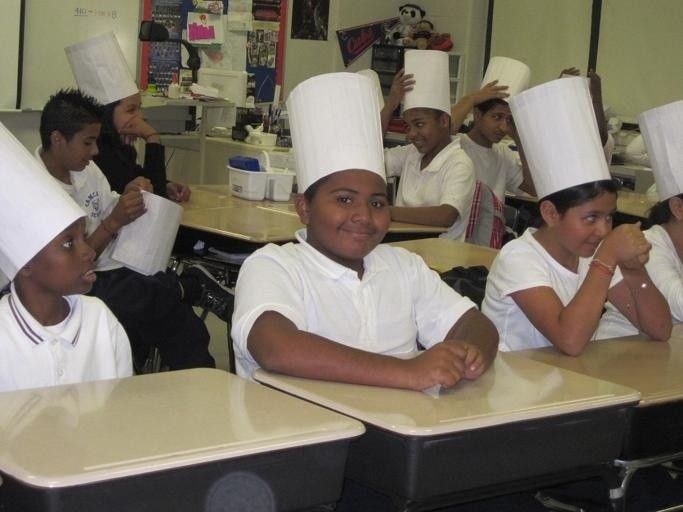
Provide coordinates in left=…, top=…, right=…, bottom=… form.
left=263, top=103, right=282, bottom=133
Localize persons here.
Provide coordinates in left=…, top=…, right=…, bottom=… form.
left=446, top=79, right=535, bottom=249
left=479, top=182, right=674, bottom=354
left=379, top=65, right=472, bottom=248
left=592, top=193, right=683, bottom=347
left=81, top=92, right=193, bottom=204
left=229, top=169, right=501, bottom=393
left=557, top=66, right=615, bottom=184
left=31, top=87, right=237, bottom=372
left=0, top=216, right=134, bottom=389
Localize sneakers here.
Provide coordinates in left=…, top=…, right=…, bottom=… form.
left=181, top=264, right=234, bottom=322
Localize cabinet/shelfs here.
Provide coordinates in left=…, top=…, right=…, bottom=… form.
left=446, top=50, right=463, bottom=107
left=367, top=43, right=419, bottom=94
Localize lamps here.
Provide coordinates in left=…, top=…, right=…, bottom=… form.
left=137, top=18, right=201, bottom=84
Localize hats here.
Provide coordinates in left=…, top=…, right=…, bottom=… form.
left=401, top=49, right=450, bottom=115
left=111, top=190, right=182, bottom=275
left=507, top=77, right=611, bottom=200
left=284, top=71, right=388, bottom=193
left=64, top=29, right=138, bottom=106
left=0, top=121, right=87, bottom=282
left=637, top=99, right=683, bottom=202
left=479, top=56, right=530, bottom=103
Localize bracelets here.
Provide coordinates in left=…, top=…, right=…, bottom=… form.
left=98, top=219, right=120, bottom=241
left=141, top=130, right=159, bottom=141
left=587, top=258, right=619, bottom=278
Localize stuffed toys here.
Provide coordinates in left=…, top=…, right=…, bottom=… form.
left=402, top=19, right=434, bottom=50
left=383, top=3, right=425, bottom=46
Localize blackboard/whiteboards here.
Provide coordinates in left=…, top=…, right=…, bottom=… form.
left=0, top=0, right=145, bottom=113
